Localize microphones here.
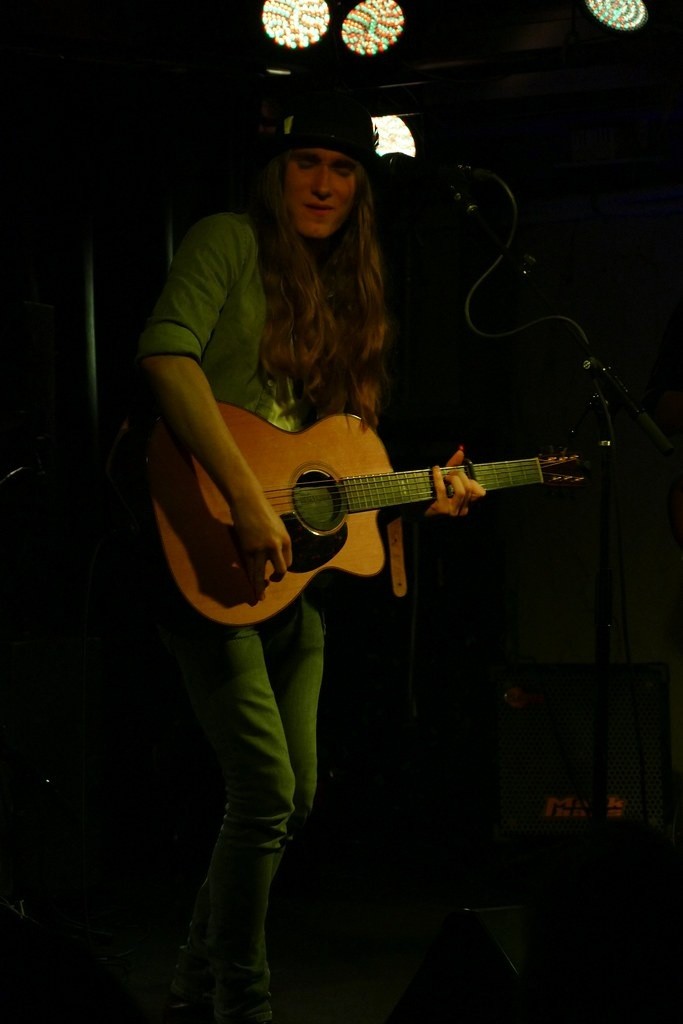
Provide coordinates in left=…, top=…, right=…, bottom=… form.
left=383, top=154, right=471, bottom=178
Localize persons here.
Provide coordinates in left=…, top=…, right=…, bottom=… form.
left=132, top=81, right=488, bottom=1023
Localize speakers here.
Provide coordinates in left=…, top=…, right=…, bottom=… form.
left=500, top=660, right=671, bottom=845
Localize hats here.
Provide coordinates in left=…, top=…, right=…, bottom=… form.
left=256, top=94, right=392, bottom=172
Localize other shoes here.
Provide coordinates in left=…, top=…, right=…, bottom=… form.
left=159, top=996, right=215, bottom=1024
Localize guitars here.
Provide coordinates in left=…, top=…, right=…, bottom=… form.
left=151, top=393, right=583, bottom=629
